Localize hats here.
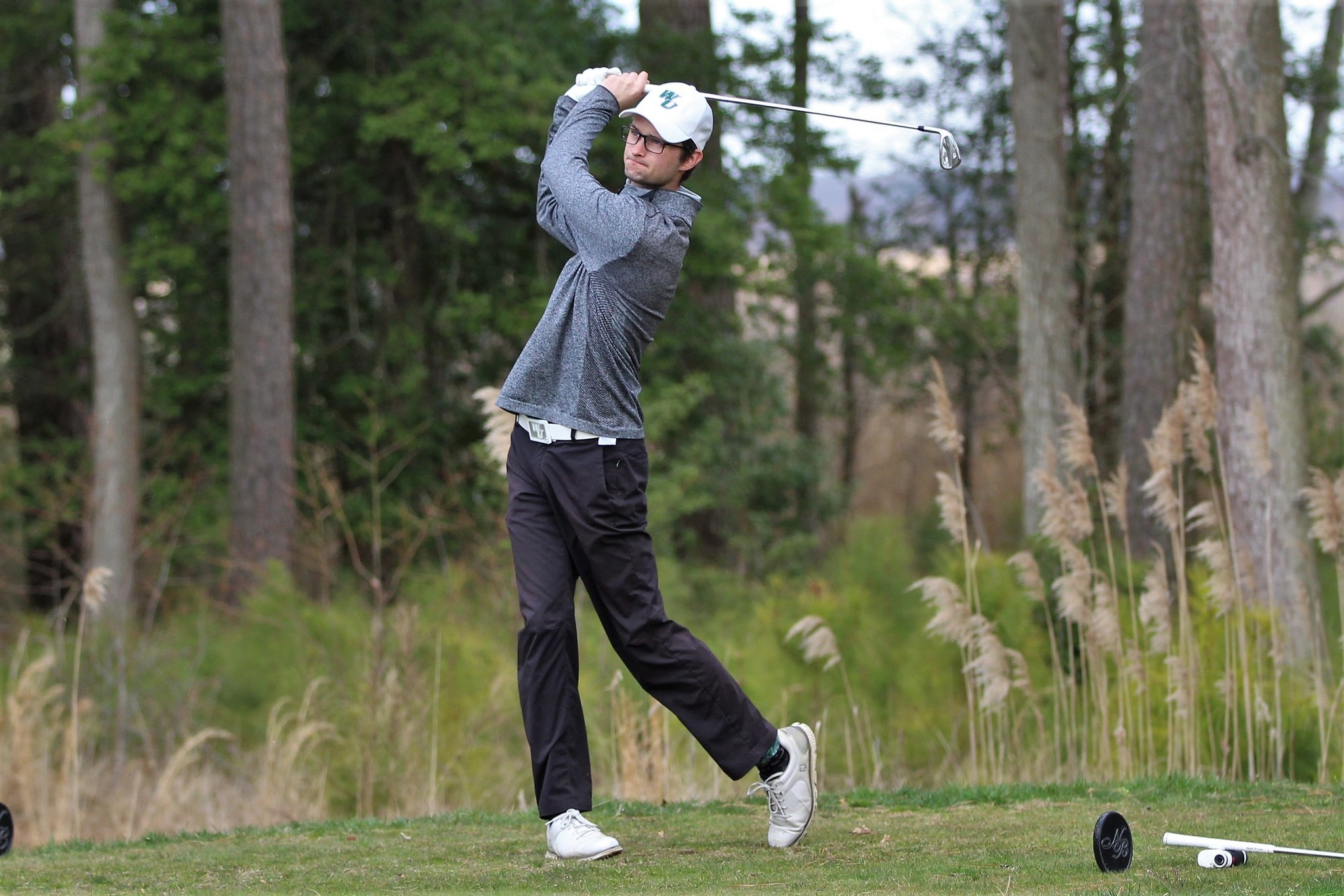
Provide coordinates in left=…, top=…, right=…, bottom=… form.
left=618, top=82, right=713, bottom=152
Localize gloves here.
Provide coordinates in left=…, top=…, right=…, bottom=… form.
left=566, top=67, right=621, bottom=102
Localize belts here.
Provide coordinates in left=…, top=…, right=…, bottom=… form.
left=518, top=413, right=600, bottom=445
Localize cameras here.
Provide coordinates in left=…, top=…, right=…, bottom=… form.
left=1197, top=850, right=1248, bottom=869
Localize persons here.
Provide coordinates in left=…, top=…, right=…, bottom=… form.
left=496, top=67, right=817, bottom=864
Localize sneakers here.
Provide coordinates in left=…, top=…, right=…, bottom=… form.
left=545, top=809, right=622, bottom=863
left=747, top=721, right=817, bottom=849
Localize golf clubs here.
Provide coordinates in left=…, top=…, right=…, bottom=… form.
left=1162, top=830, right=1343, bottom=862
left=607, top=73, right=964, bottom=170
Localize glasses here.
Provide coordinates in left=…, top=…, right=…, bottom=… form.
left=621, top=126, right=686, bottom=154
